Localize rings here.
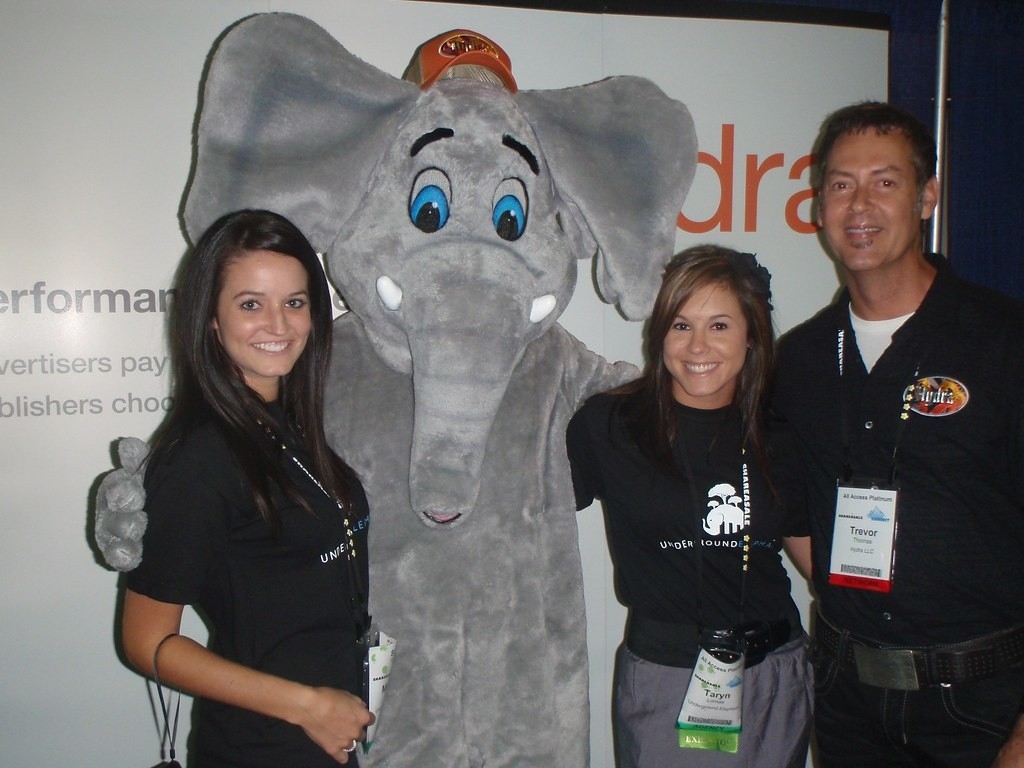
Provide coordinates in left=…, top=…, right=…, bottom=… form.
left=342, top=740, right=357, bottom=752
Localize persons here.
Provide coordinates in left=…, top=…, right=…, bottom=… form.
left=565, top=244, right=813, bottom=768
left=765, top=100, right=1024, bottom=768
left=122, top=209, right=377, bottom=768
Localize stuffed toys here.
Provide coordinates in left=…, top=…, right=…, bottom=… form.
left=93, top=9, right=699, bottom=768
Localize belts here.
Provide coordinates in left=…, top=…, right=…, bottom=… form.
left=816, top=604, right=1024, bottom=691
